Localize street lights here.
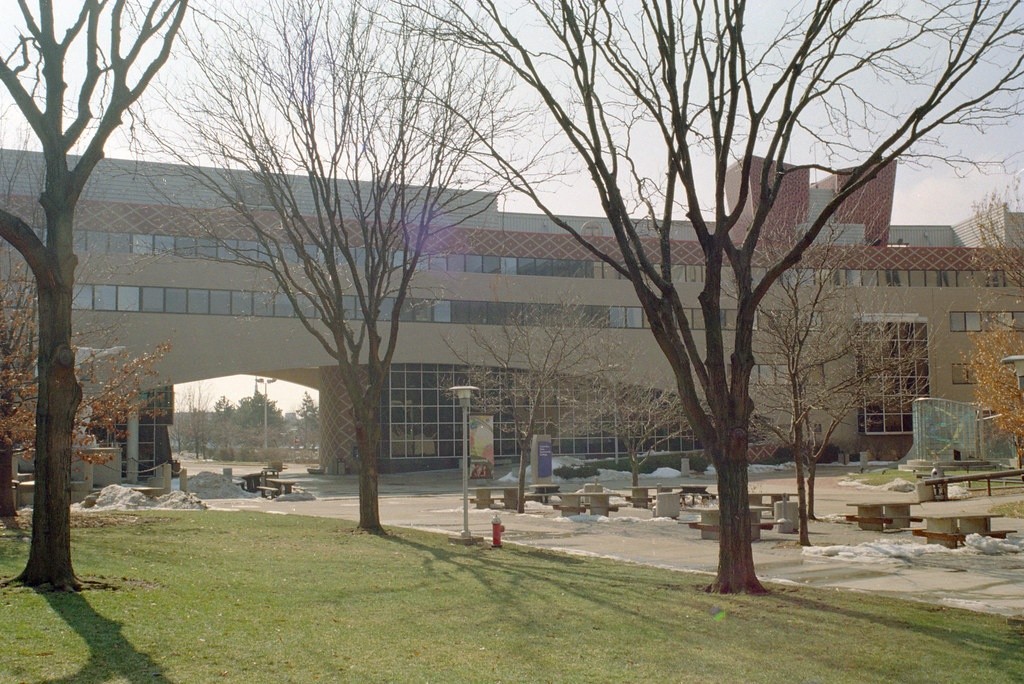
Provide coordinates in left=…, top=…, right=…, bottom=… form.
left=256, top=378, right=277, bottom=448
left=450, top=385, right=480, bottom=537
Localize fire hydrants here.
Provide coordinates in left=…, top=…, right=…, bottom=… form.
left=491, top=514, right=505, bottom=548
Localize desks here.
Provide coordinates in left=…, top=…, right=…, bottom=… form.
left=748, top=493, right=799, bottom=516
left=270, top=479, right=294, bottom=497
left=910, top=514, right=1004, bottom=549
left=846, top=501, right=920, bottom=532
left=683, top=505, right=772, bottom=540
left=466, top=484, right=714, bottom=517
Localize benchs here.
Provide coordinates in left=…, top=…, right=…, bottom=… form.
left=836, top=514, right=924, bottom=532
left=677, top=503, right=784, bottom=532
left=257, top=486, right=278, bottom=499
left=900, top=527, right=1018, bottom=548
left=458, top=493, right=717, bottom=517
